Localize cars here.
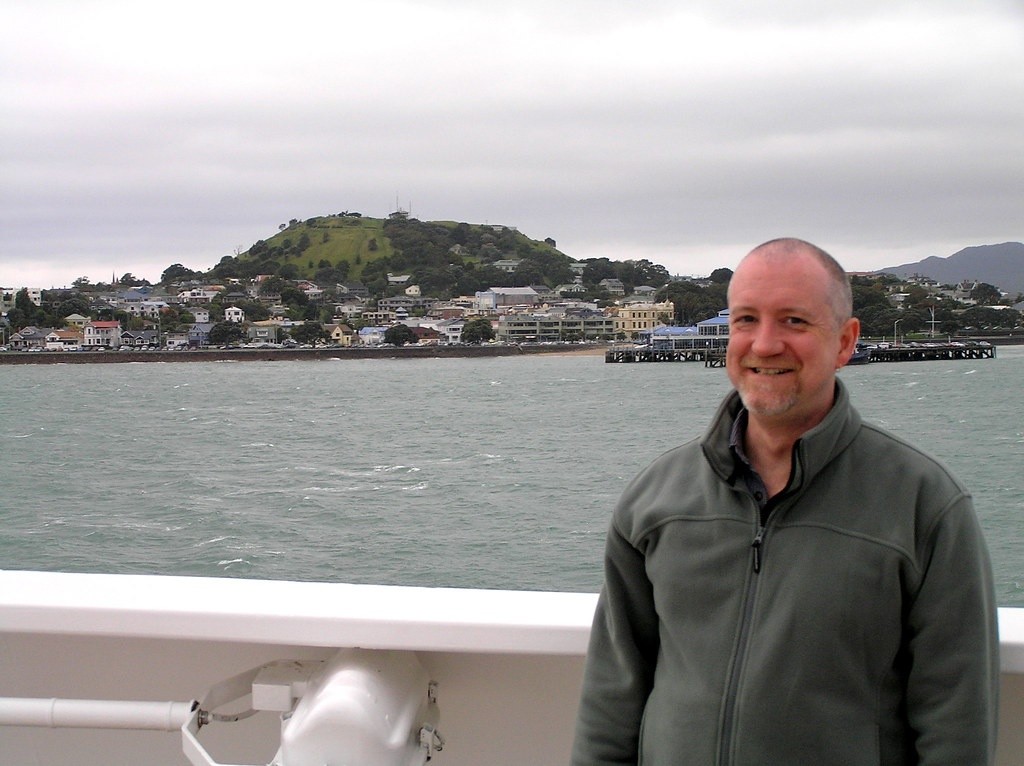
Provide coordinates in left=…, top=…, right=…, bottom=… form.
left=965, top=326, right=1024, bottom=330
left=0, top=339, right=618, bottom=351
left=857, top=341, right=990, bottom=349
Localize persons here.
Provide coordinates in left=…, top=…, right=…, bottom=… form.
left=569, top=238, right=1000, bottom=766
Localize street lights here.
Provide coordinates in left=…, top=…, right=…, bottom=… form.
left=894, top=319, right=903, bottom=347
left=158, top=318, right=161, bottom=347
left=7, top=319, right=10, bottom=351
